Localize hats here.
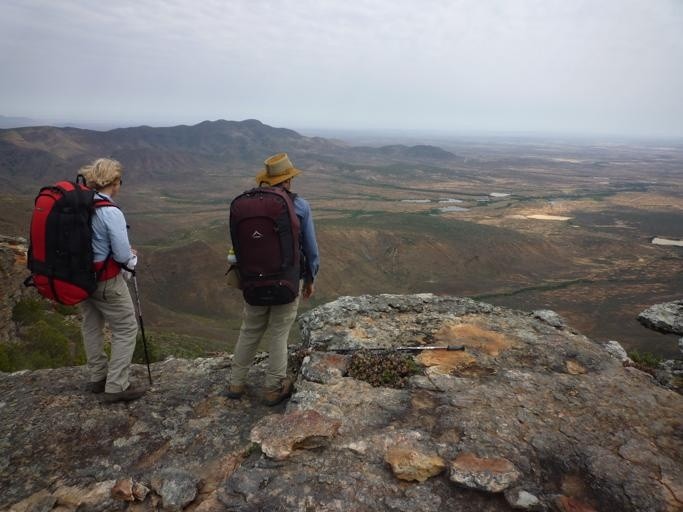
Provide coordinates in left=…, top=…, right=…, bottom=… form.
left=256, top=153, right=301, bottom=187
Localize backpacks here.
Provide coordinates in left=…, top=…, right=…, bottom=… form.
left=26, top=174, right=120, bottom=305
left=228, top=180, right=302, bottom=307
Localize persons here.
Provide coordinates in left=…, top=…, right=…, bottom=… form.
left=76, top=159, right=147, bottom=404
left=225, top=153, right=319, bottom=406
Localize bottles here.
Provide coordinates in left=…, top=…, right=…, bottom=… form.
left=227, top=249, right=237, bottom=265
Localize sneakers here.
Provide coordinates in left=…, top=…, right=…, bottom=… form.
left=105, top=382, right=146, bottom=404
left=92, top=378, right=105, bottom=393
left=264, top=380, right=291, bottom=405
left=228, top=385, right=245, bottom=398
